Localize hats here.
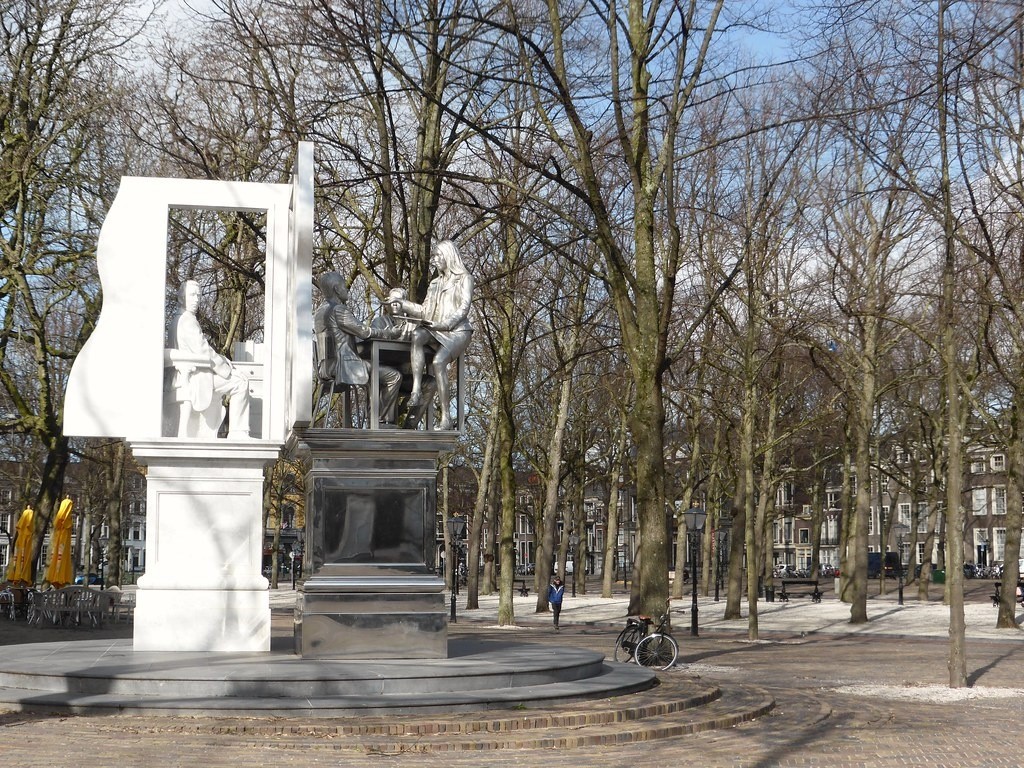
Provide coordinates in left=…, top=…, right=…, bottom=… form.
left=554, top=576, right=560, bottom=580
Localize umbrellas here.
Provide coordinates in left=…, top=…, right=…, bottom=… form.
left=7, top=499, right=74, bottom=588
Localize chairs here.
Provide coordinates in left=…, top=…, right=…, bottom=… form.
left=161, top=348, right=211, bottom=438
left=308, top=329, right=372, bottom=429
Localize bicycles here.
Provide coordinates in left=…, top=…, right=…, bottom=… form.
left=613, top=595, right=685, bottom=672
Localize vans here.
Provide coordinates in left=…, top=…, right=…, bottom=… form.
left=555, top=561, right=589, bottom=575
left=868, top=552, right=901, bottom=580
left=773, top=563, right=797, bottom=578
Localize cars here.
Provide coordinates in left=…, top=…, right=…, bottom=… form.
left=801, top=562, right=833, bottom=578
left=517, top=562, right=535, bottom=575
left=963, top=559, right=1024, bottom=581
left=75, top=572, right=98, bottom=586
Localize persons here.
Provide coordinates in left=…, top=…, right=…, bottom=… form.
left=546, top=576, right=566, bottom=629
left=993, top=564, right=1001, bottom=579
left=314, top=240, right=475, bottom=430
left=771, top=565, right=841, bottom=578
left=167, top=280, right=251, bottom=444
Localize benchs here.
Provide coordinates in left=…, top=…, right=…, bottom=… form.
left=774, top=578, right=823, bottom=603
left=0, top=584, right=136, bottom=630
left=512, top=579, right=530, bottom=598
left=988, top=581, right=1024, bottom=607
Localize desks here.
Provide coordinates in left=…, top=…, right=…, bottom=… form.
left=342, top=337, right=465, bottom=436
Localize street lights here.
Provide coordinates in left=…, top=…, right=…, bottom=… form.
left=289, top=540, right=299, bottom=591
left=682, top=499, right=708, bottom=639
left=711, top=525, right=728, bottom=601
left=446, top=511, right=466, bottom=624
left=622, top=543, right=631, bottom=589
left=893, top=522, right=910, bottom=605
left=569, top=531, right=580, bottom=598
left=130, top=546, right=139, bottom=584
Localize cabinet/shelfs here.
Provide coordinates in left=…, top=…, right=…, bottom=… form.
left=229, top=361, right=264, bottom=438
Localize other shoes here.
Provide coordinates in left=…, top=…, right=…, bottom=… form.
left=555, top=629, right=559, bottom=634
left=553, top=624, right=559, bottom=629
